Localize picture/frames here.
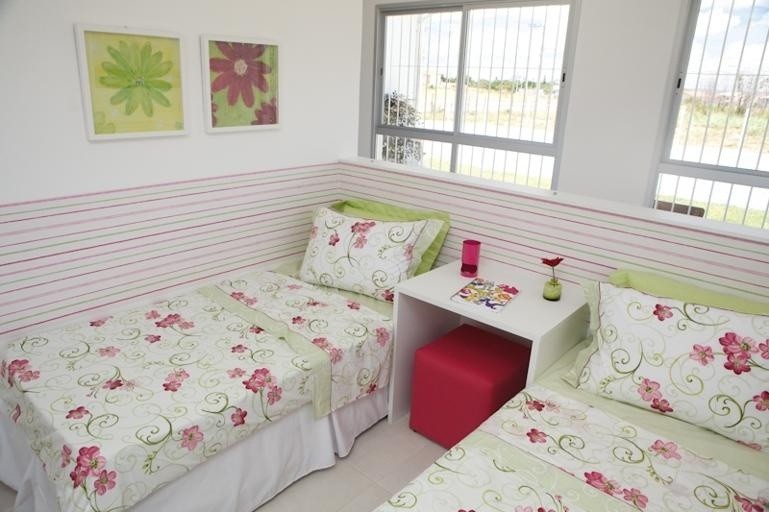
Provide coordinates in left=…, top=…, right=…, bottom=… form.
left=75, top=28, right=283, bottom=141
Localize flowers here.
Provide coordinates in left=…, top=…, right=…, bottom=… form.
left=541, top=255, right=564, bottom=280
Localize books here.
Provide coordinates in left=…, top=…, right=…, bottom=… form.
left=450, top=277, right=521, bottom=314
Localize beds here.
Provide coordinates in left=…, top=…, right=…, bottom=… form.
left=0, top=253, right=390, bottom=512
left=370, top=302, right=769, bottom=512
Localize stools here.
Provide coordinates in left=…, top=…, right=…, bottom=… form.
left=409, top=324, right=530, bottom=450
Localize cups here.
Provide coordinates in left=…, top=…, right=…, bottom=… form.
left=459, top=240, right=481, bottom=278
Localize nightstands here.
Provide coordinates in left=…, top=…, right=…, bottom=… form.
left=385, top=258, right=591, bottom=427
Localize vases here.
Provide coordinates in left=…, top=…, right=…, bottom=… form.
left=542, top=278, right=562, bottom=301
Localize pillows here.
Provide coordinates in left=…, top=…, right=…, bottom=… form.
left=297, top=195, right=448, bottom=307
left=560, top=269, right=769, bottom=455
